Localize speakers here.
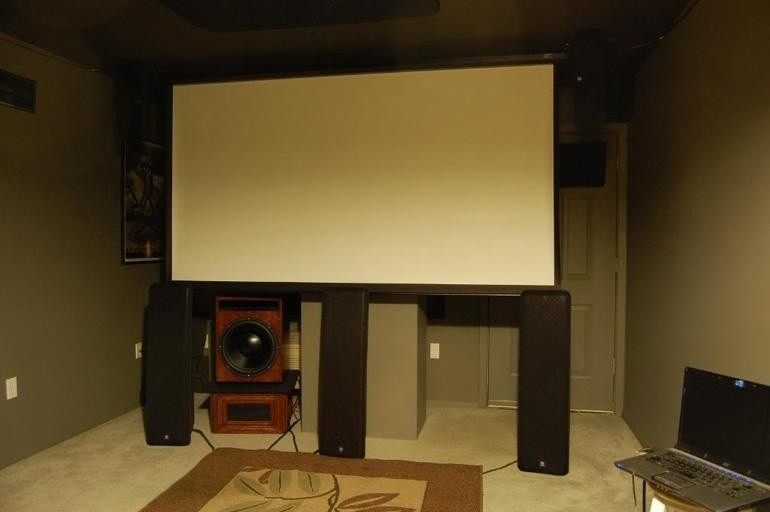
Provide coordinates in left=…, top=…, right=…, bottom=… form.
left=555, top=139, right=609, bottom=189
left=140, top=281, right=193, bottom=446
left=212, top=294, right=283, bottom=384
left=315, top=287, right=369, bottom=458
left=572, top=53, right=636, bottom=124
left=517, top=290, right=572, bottom=476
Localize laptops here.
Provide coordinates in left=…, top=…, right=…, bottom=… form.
left=612, top=365, right=769, bottom=512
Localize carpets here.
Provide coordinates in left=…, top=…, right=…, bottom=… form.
left=134, top=446, right=484, bottom=512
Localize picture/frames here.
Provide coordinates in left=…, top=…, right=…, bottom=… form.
left=120, top=137, right=169, bottom=264
